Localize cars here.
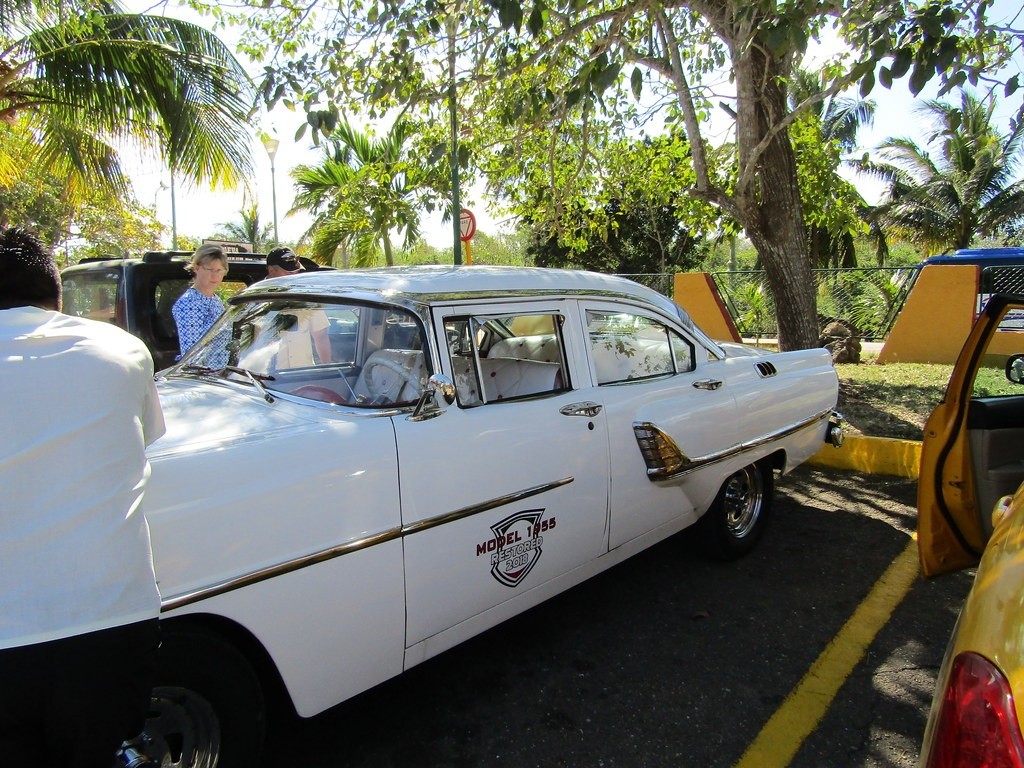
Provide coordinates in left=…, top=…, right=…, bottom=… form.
left=917, top=291, right=1024, bottom=768
left=115, top=265, right=840, bottom=768
left=56, top=252, right=339, bottom=376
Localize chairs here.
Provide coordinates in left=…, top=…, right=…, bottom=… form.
left=347, top=347, right=428, bottom=409
left=455, top=357, right=564, bottom=407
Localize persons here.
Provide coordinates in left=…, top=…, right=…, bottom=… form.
left=173, top=245, right=235, bottom=370
left=0, top=227, right=167, bottom=768
left=266, top=247, right=332, bottom=369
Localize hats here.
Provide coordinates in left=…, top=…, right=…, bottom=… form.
left=267, top=246, right=306, bottom=272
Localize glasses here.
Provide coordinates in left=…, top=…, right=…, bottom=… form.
left=202, top=266, right=226, bottom=275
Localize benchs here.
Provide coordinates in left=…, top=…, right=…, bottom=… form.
left=485, top=333, right=688, bottom=403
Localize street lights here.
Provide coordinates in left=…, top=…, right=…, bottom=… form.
left=263, top=125, right=279, bottom=249
left=154, top=180, right=169, bottom=214
